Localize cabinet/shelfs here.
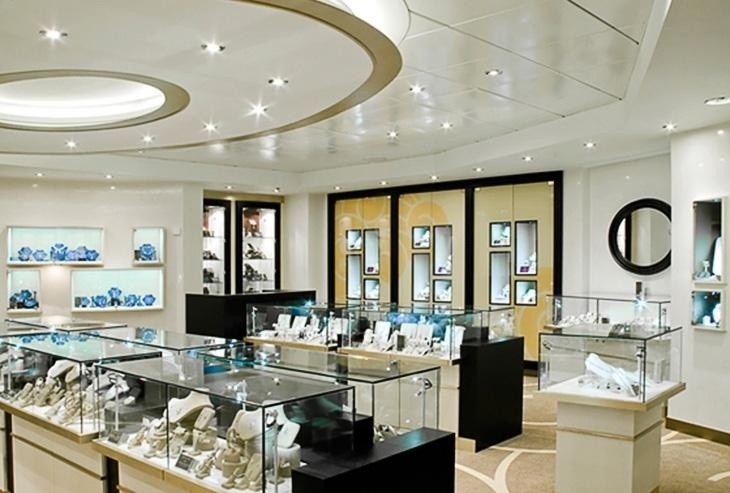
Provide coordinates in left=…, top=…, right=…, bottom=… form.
left=3, top=226, right=166, bottom=317
left=411, top=225, right=453, bottom=303
left=690, top=200, right=724, bottom=330
left=345, top=228, right=380, bottom=300
left=203, top=198, right=231, bottom=294
left=488, top=220, right=537, bottom=306
left=235, top=200, right=280, bottom=292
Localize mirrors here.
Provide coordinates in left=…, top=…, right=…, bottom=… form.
left=608, top=197, right=671, bottom=276
